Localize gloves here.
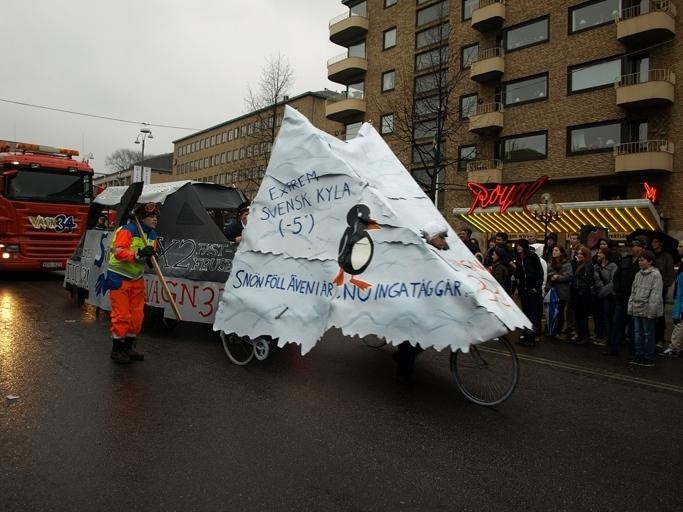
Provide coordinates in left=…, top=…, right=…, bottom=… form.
left=138, top=246, right=157, bottom=269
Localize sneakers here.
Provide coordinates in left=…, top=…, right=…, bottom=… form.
left=558, top=330, right=611, bottom=346
left=630, top=342, right=678, bottom=367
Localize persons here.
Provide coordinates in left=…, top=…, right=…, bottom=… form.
left=106, top=201, right=160, bottom=364
left=96, top=217, right=106, bottom=228
left=459, top=229, right=683, bottom=367
left=225, top=200, right=252, bottom=243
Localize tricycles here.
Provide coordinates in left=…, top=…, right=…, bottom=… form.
left=210, top=104, right=533, bottom=408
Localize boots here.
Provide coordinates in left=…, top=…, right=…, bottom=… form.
left=111, top=336, right=143, bottom=364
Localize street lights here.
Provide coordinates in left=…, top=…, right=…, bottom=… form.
left=135, top=127, right=153, bottom=182
left=80, top=152, right=96, bottom=168
left=532, top=193, right=563, bottom=240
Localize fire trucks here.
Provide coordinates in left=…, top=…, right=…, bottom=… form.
left=1, top=137, right=107, bottom=272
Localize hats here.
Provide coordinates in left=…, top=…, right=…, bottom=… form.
left=136, top=203, right=160, bottom=219
